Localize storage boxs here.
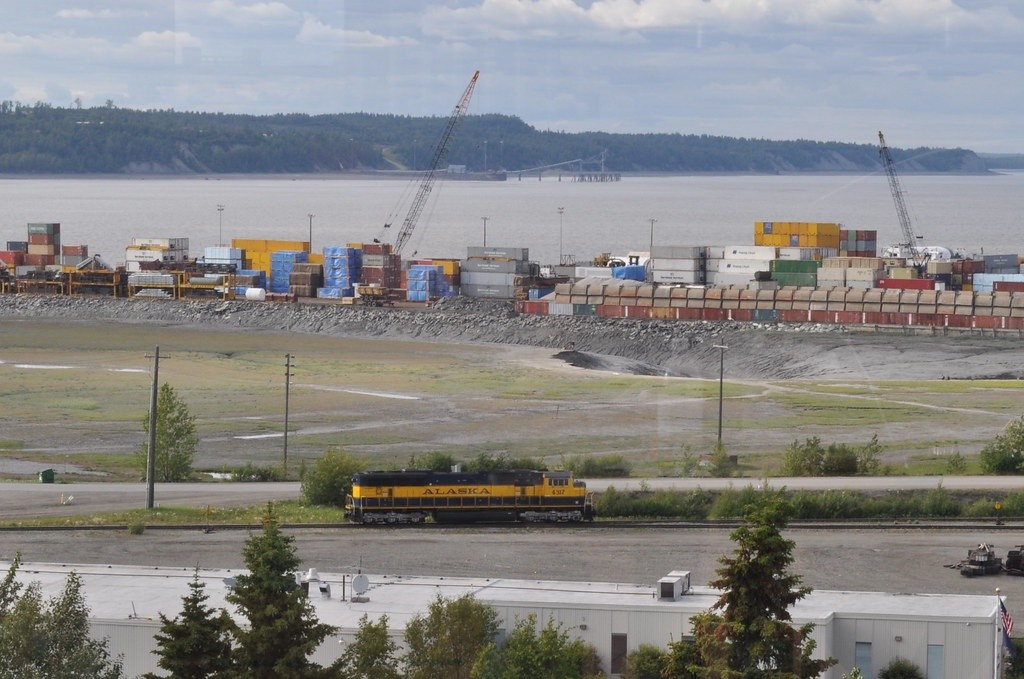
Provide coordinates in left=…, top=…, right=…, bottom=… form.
left=0, top=221, right=1024, bottom=330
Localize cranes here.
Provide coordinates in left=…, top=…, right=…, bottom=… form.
left=876, top=130, right=932, bottom=279
left=367, top=69, right=480, bottom=256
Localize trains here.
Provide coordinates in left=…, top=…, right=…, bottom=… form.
left=342, top=466, right=597, bottom=526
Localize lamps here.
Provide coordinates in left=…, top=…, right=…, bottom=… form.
left=895, top=636, right=902, bottom=641
left=580, top=624, right=586, bottom=630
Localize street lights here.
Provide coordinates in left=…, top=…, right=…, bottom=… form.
left=217, top=204, right=226, bottom=247
left=649, top=217, right=657, bottom=247
left=308, top=213, right=316, bottom=253
left=481, top=215, right=489, bottom=247
left=558, top=206, right=566, bottom=266
